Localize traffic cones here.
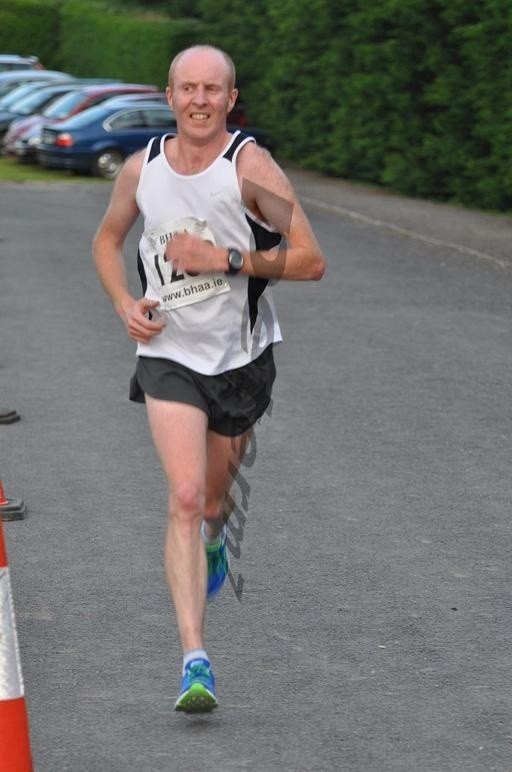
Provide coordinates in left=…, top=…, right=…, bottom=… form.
left=0, top=480, right=27, bottom=521
left=0, top=509, right=34, bottom=772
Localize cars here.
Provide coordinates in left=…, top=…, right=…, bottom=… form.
left=0, top=53, right=274, bottom=180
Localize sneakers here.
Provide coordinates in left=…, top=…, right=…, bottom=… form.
left=173, top=657, right=219, bottom=721
left=201, top=520, right=232, bottom=600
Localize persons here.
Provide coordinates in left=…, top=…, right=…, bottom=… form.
left=90, top=46, right=326, bottom=714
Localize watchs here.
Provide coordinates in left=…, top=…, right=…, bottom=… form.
left=225, top=248, right=245, bottom=274
